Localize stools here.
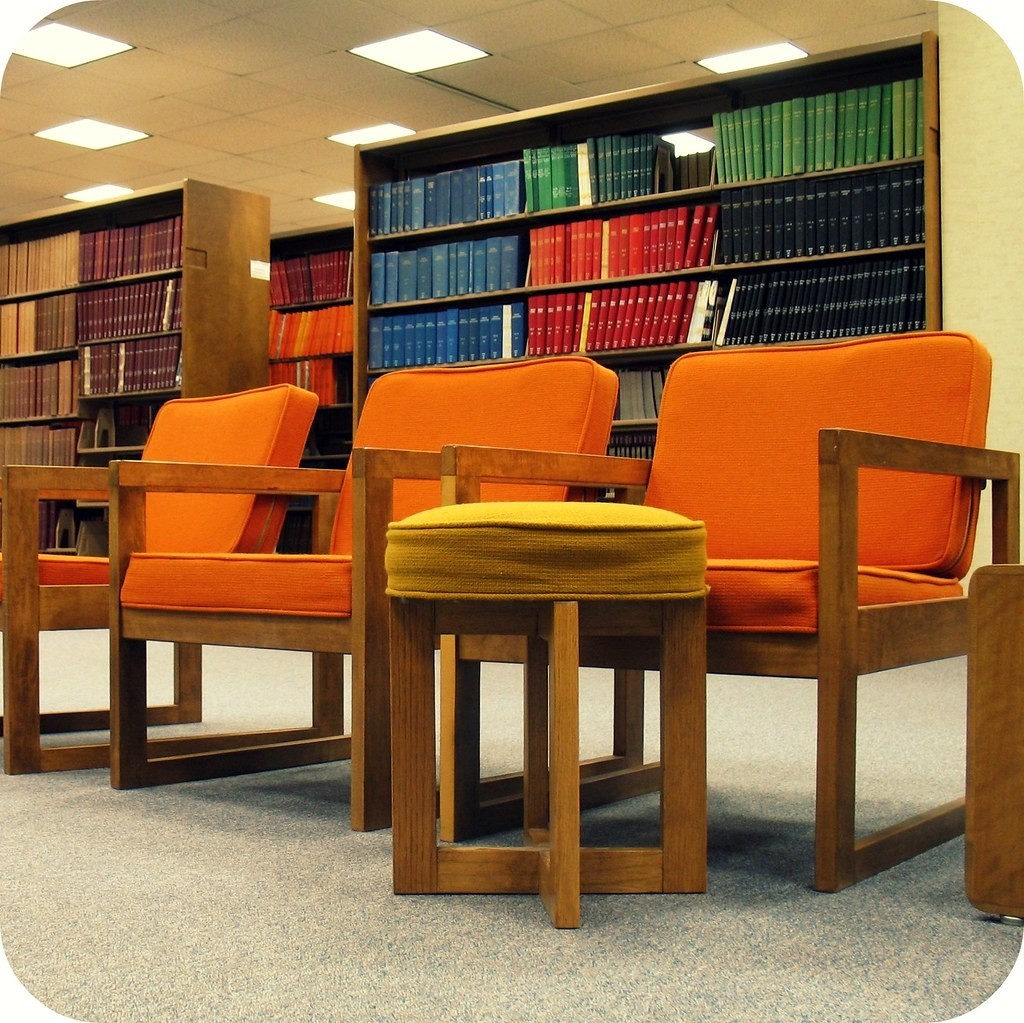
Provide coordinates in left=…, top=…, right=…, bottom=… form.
left=384, top=499, right=707, bottom=895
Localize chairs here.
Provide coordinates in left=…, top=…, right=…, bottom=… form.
left=108, top=354, right=620, bottom=832
left=440, top=329, right=1019, bottom=892
left=0, top=382, right=345, bottom=790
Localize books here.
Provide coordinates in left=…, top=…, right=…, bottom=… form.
left=0, top=211, right=355, bottom=554
left=367, top=75, right=923, bottom=494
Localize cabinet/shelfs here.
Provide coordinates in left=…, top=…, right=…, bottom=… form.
left=351, top=29, right=939, bottom=434
left=270, top=221, right=354, bottom=514
left=0, top=178, right=271, bottom=553
